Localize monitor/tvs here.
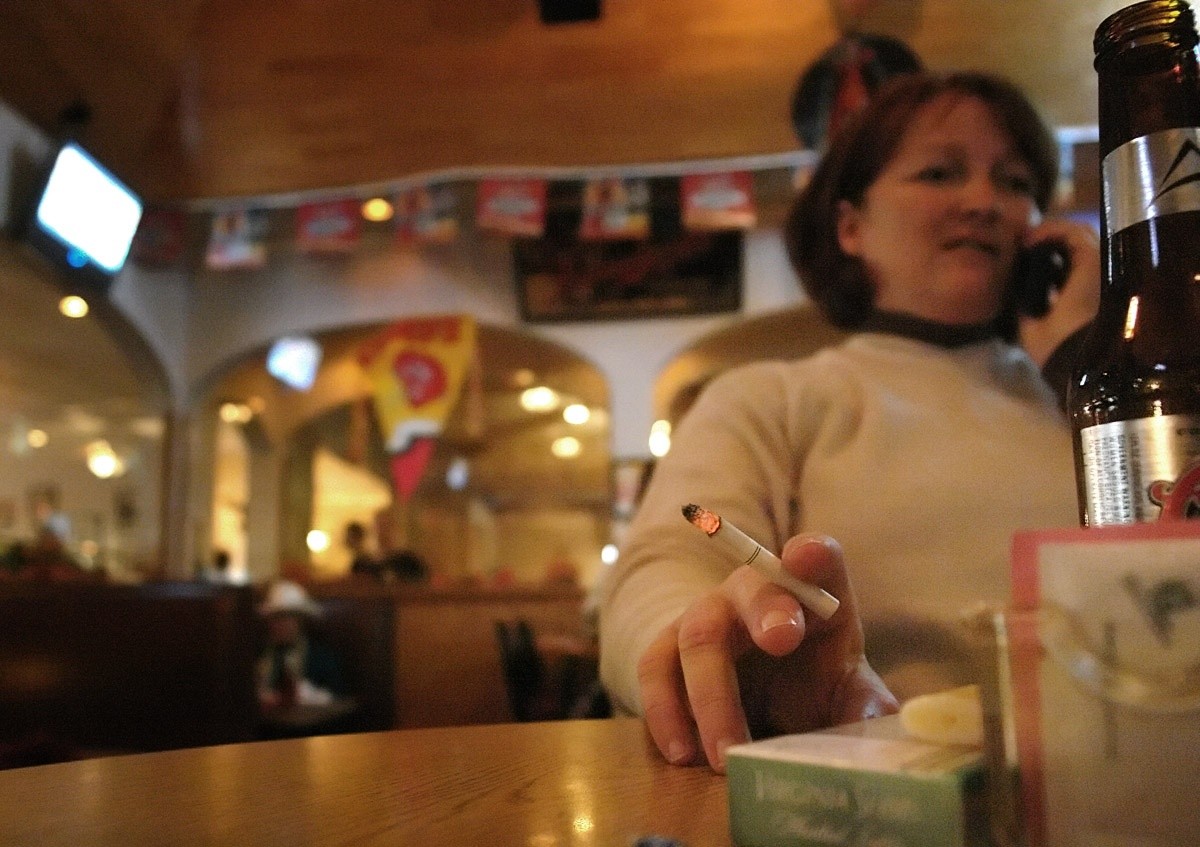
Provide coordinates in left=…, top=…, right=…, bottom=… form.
left=26, top=144, right=144, bottom=294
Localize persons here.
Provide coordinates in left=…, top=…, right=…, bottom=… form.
left=341, top=522, right=382, bottom=582
left=594, top=66, right=1114, bottom=772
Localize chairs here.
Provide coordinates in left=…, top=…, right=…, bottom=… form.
left=497, top=622, right=617, bottom=720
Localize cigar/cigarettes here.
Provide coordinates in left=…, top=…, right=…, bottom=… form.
left=680, top=503, right=840, bottom=623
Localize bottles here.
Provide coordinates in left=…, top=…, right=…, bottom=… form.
left=1069, top=1, right=1200, bottom=527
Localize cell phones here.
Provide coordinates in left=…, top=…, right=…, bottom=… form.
left=1017, top=244, right=1048, bottom=317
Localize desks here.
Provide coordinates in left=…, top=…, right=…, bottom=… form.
left=318, top=590, right=585, bottom=732
left=0, top=717, right=731, bottom=847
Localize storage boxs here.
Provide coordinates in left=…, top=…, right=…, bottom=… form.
left=725, top=714, right=993, bottom=846
